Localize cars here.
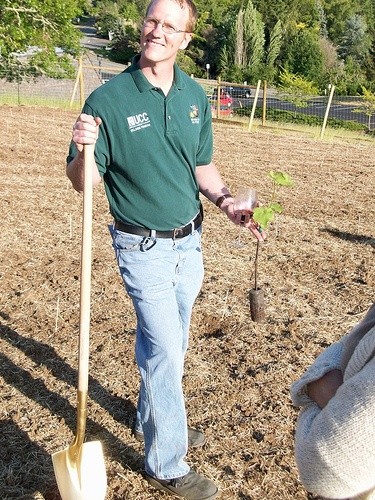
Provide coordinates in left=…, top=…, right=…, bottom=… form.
left=215, top=84, right=252, bottom=99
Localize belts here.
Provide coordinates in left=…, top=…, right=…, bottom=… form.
left=112, top=208, right=205, bottom=240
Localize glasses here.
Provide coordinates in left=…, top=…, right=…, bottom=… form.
left=142, top=16, right=189, bottom=36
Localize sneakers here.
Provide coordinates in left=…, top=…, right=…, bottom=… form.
left=144, top=463, right=219, bottom=500
left=134, top=423, right=207, bottom=447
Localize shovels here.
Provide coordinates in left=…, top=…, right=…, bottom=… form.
left=50, top=145, right=108, bottom=499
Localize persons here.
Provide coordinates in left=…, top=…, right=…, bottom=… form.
left=290, top=301, right=375, bottom=500
left=66, top=0, right=261, bottom=500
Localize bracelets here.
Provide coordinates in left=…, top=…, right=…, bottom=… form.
left=215, top=194, right=232, bottom=208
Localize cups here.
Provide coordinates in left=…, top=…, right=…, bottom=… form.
left=233, top=188, right=259, bottom=223
